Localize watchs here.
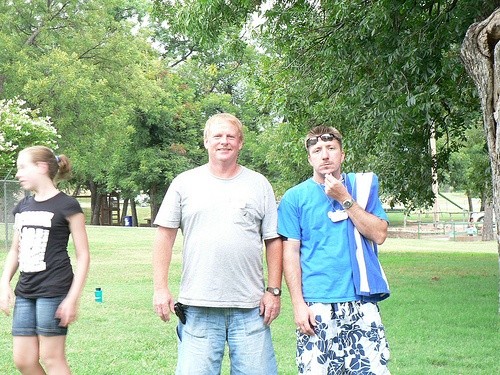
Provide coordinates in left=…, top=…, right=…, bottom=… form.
left=342, top=198, right=353, bottom=209
left=266, top=287, right=281, bottom=296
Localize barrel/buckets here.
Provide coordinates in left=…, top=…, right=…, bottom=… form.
left=124, top=216, right=132, bottom=226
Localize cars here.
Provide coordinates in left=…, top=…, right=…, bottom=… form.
left=469, top=201, right=497, bottom=230
left=118, top=190, right=150, bottom=204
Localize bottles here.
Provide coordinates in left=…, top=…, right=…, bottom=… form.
left=95, top=288, right=102, bottom=302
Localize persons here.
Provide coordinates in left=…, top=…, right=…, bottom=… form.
left=467, top=218, right=477, bottom=236
left=276, top=125, right=392, bottom=375
left=0, top=146, right=91, bottom=375
left=151, top=112, right=283, bottom=375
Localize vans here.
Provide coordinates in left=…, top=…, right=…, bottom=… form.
left=381, top=196, right=419, bottom=212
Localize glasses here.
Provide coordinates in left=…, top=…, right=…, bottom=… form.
left=307, top=133, right=340, bottom=148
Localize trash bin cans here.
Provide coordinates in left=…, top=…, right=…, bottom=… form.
left=124, top=216, right=132, bottom=226
